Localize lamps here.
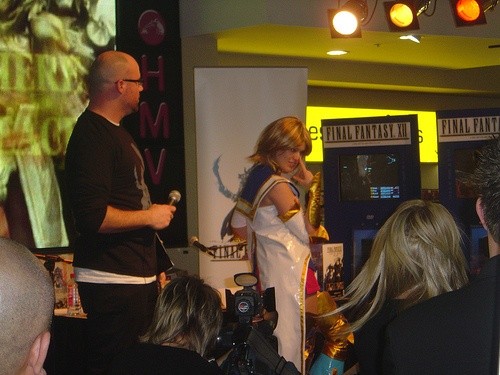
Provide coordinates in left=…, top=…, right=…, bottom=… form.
left=451, top=0, right=496, bottom=29
left=382, top=0, right=430, bottom=33
left=325, top=0, right=369, bottom=39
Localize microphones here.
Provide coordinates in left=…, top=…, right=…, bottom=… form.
left=190, top=236, right=207, bottom=252
left=167, top=190, right=181, bottom=205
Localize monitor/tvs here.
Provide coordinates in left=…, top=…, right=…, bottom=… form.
left=339, top=152, right=401, bottom=200
left=453, top=147, right=483, bottom=198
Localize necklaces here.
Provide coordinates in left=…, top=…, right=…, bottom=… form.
left=161, top=337, right=184, bottom=347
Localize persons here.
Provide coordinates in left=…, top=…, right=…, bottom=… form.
left=381, top=133, right=500, bottom=375
left=107, top=277, right=226, bottom=375
left=313, top=199, right=471, bottom=375
left=311, top=214, right=329, bottom=243
left=0, top=236, right=55, bottom=375
left=235, top=116, right=352, bottom=375
left=64, top=51, right=176, bottom=375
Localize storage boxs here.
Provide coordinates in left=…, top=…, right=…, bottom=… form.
left=312, top=242, right=343, bottom=296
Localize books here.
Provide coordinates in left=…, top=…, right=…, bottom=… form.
left=311, top=243, right=343, bottom=292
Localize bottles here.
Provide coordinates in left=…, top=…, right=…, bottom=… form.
left=54, top=276, right=66, bottom=308
left=335, top=265, right=341, bottom=290
left=67, top=274, right=78, bottom=308
left=327, top=266, right=336, bottom=291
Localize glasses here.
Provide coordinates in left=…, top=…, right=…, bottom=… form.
left=115, top=79, right=144, bottom=85
